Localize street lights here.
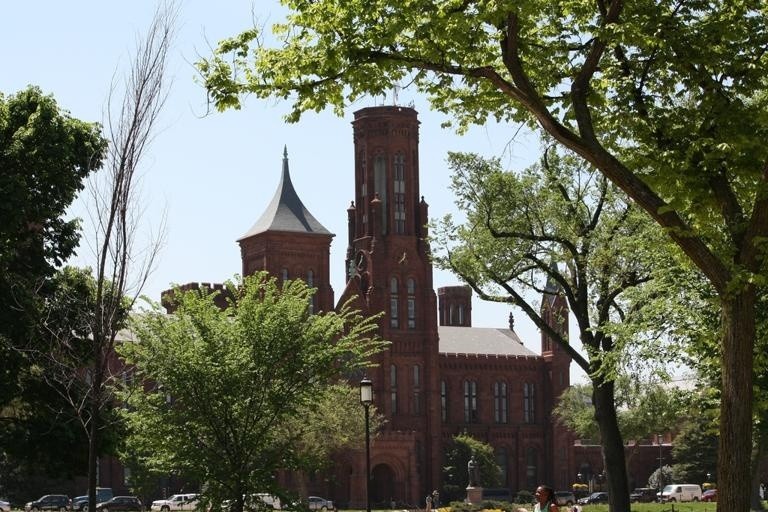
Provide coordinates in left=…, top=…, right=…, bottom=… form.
left=656, top=433, right=665, bottom=505
left=358, top=374, right=376, bottom=511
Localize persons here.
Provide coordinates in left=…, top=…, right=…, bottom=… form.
left=432, top=495, right=440, bottom=508
left=533, top=484, right=559, bottom=512
left=425, top=494, right=432, bottom=511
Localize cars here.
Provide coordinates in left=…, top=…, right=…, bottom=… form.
left=479, top=480, right=719, bottom=505
